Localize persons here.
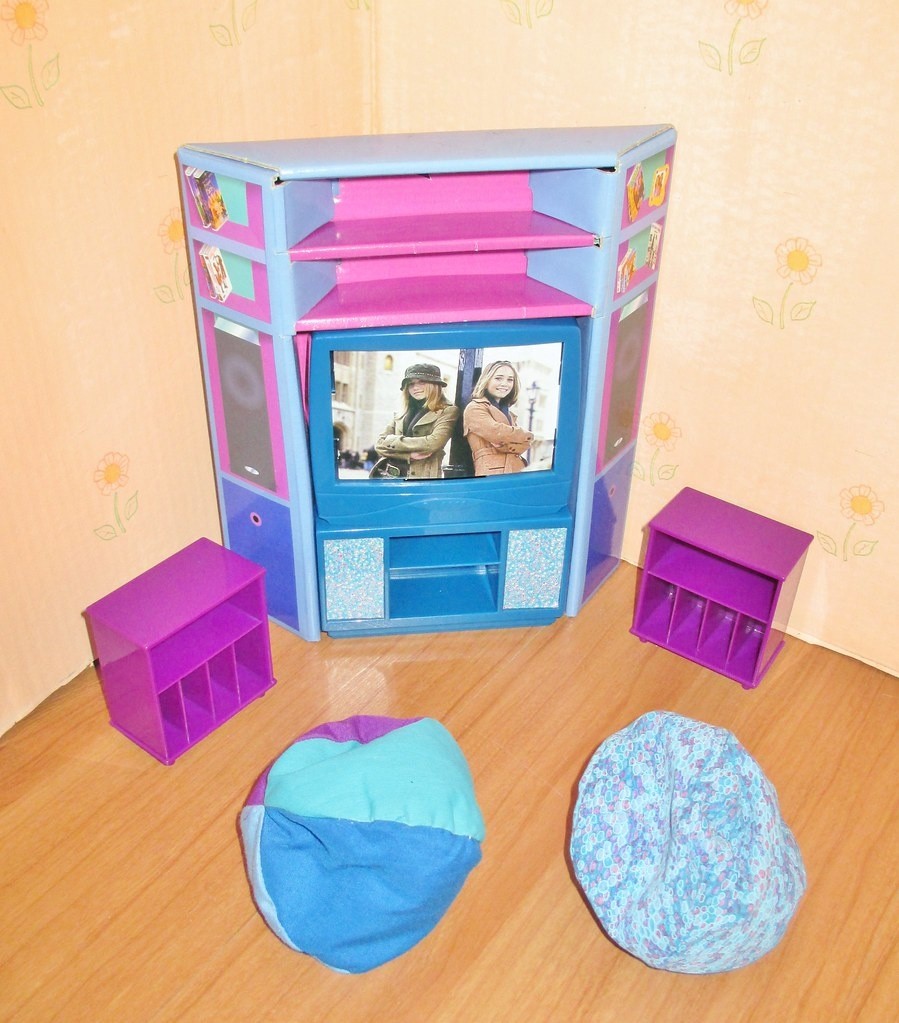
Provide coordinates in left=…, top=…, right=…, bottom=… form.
left=368, top=363, right=461, bottom=480
left=337, top=445, right=381, bottom=472
left=462, top=359, right=535, bottom=477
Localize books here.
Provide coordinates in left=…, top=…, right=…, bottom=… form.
left=616, top=248, right=638, bottom=295
left=644, top=221, right=662, bottom=270
left=184, top=165, right=230, bottom=232
left=625, top=161, right=645, bottom=222
left=197, top=243, right=233, bottom=304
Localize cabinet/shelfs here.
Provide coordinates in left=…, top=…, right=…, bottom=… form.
left=317, top=508, right=575, bottom=638
left=287, top=180, right=601, bottom=333
left=630, top=488, right=814, bottom=690
left=81, top=538, right=278, bottom=767
